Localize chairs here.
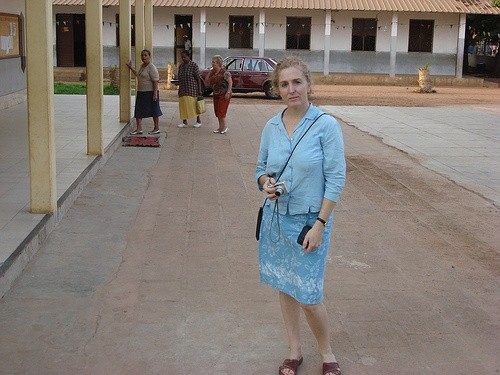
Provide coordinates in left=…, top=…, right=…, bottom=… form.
left=248, top=63, right=251, bottom=71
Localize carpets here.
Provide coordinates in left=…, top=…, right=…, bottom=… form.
left=122, top=136, right=161, bottom=148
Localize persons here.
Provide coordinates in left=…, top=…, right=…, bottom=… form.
left=467, top=41, right=475, bottom=53
left=257, top=57, right=346, bottom=375
left=208, top=55, right=233, bottom=133
left=126, top=49, right=163, bottom=135
left=177, top=50, right=202, bottom=128
left=182, top=34, right=191, bottom=57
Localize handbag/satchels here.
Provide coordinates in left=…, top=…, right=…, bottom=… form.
left=254, top=207, right=264, bottom=241
left=196, top=98, right=207, bottom=115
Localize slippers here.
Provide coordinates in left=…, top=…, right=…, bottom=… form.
left=279, top=356, right=303, bottom=375
left=321, top=361, right=341, bottom=375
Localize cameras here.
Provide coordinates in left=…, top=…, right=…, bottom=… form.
left=267, top=181, right=290, bottom=199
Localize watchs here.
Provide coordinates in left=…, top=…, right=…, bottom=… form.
left=317, top=218, right=327, bottom=225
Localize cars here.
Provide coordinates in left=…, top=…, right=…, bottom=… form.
left=171, top=56, right=282, bottom=100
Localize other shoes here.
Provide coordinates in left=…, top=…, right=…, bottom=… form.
left=221, top=126, right=229, bottom=134
left=148, top=128, right=161, bottom=135
left=213, top=130, right=222, bottom=133
left=193, top=122, right=202, bottom=128
left=177, top=123, right=189, bottom=128
left=129, top=128, right=144, bottom=134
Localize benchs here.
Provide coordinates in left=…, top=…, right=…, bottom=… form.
left=258, top=62, right=266, bottom=71
